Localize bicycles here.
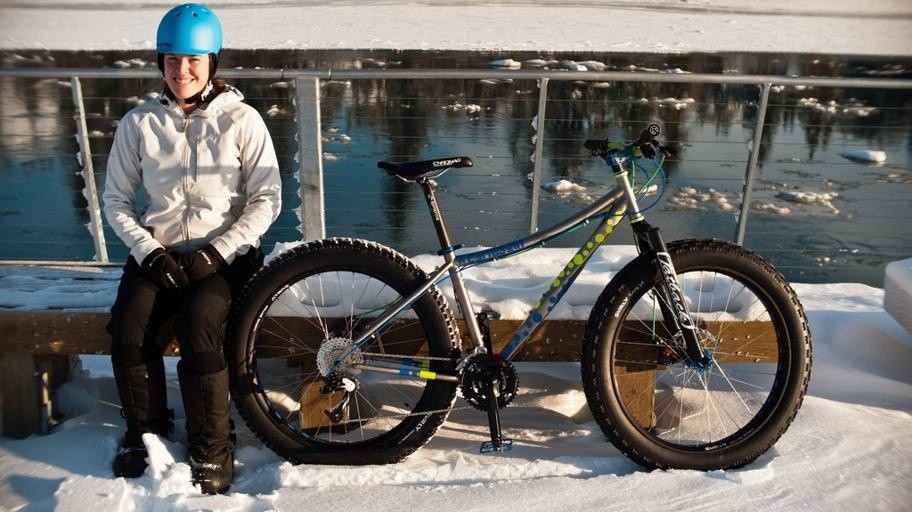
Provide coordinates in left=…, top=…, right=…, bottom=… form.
left=226, top=123, right=814, bottom=472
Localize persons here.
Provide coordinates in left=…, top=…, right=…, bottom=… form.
left=100, top=4, right=282, bottom=492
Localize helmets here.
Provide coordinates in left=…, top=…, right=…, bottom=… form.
left=157, top=3, right=222, bottom=67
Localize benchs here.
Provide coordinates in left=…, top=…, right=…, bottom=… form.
left=0, top=260, right=783, bottom=442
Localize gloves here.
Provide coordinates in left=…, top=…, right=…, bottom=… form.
left=146, top=248, right=190, bottom=289
left=184, top=246, right=225, bottom=282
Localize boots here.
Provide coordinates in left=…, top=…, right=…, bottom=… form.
left=110, top=354, right=171, bottom=480
left=177, top=359, right=237, bottom=495
